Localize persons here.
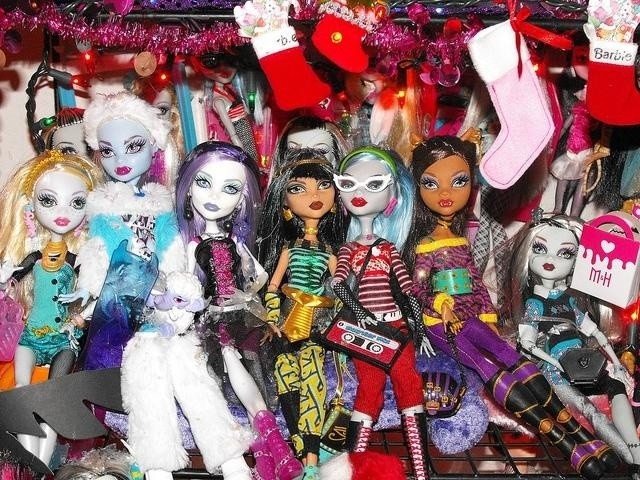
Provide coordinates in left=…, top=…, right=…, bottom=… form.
left=0, top=92, right=640, bottom=480
